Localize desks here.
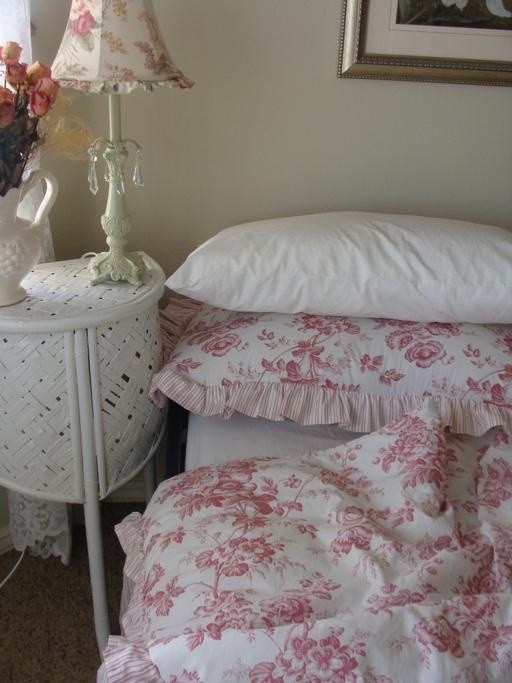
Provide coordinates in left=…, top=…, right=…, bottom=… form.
left=0, top=246, right=168, bottom=661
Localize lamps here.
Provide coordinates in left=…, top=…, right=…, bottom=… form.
left=50, top=0, right=197, bottom=288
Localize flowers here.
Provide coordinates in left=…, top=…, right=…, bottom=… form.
left=1, top=39, right=98, bottom=199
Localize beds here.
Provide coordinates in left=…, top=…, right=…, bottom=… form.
left=182, top=413, right=510, bottom=683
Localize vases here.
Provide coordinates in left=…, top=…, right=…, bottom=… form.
left=0, top=167, right=61, bottom=309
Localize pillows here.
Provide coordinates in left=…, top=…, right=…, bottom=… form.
left=149, top=304, right=512, bottom=437
left=165, top=209, right=512, bottom=317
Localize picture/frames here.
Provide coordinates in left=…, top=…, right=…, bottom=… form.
left=335, top=1, right=511, bottom=86
